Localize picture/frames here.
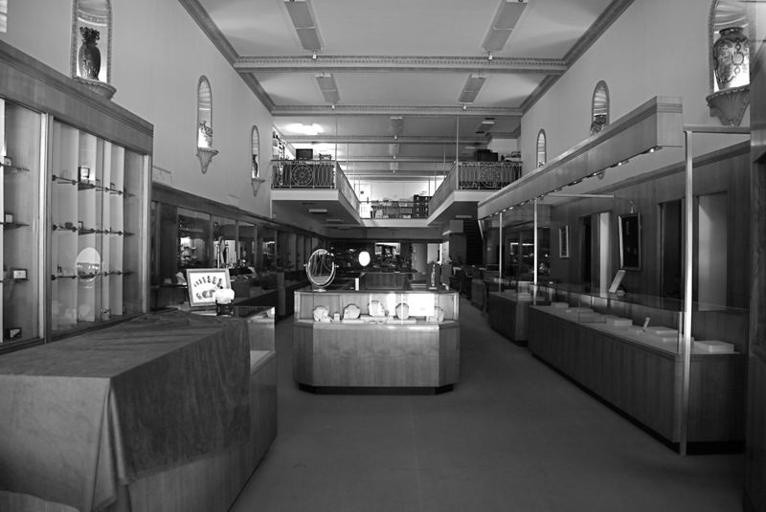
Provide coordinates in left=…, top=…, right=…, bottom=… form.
left=617, top=213, right=641, bottom=272
left=559, top=225, right=569, bottom=259
left=187, top=268, right=232, bottom=307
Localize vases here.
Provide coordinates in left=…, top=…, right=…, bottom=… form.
left=714, top=27, right=751, bottom=89
left=79, top=26, right=101, bottom=81
left=253, top=154, right=258, bottom=177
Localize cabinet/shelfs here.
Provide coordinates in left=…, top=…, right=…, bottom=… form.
left=0, top=41, right=152, bottom=353
